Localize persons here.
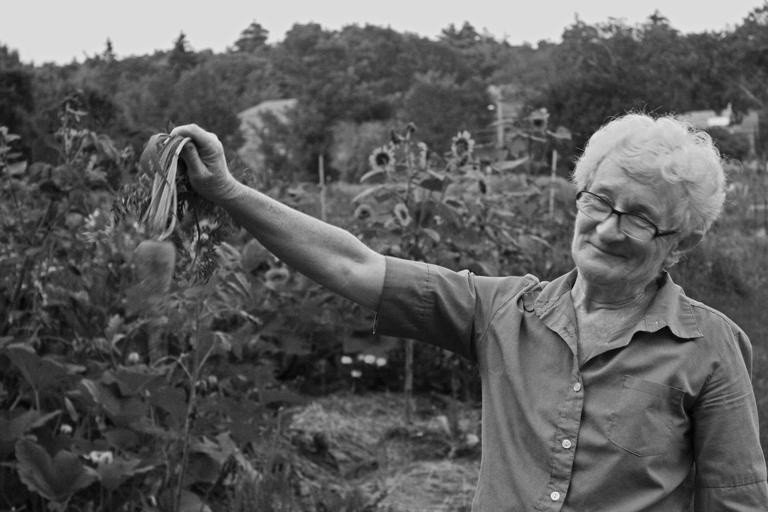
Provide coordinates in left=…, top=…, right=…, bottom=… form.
left=170, top=112, right=768, bottom=512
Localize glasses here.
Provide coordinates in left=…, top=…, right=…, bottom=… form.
left=576, top=186, right=676, bottom=241
